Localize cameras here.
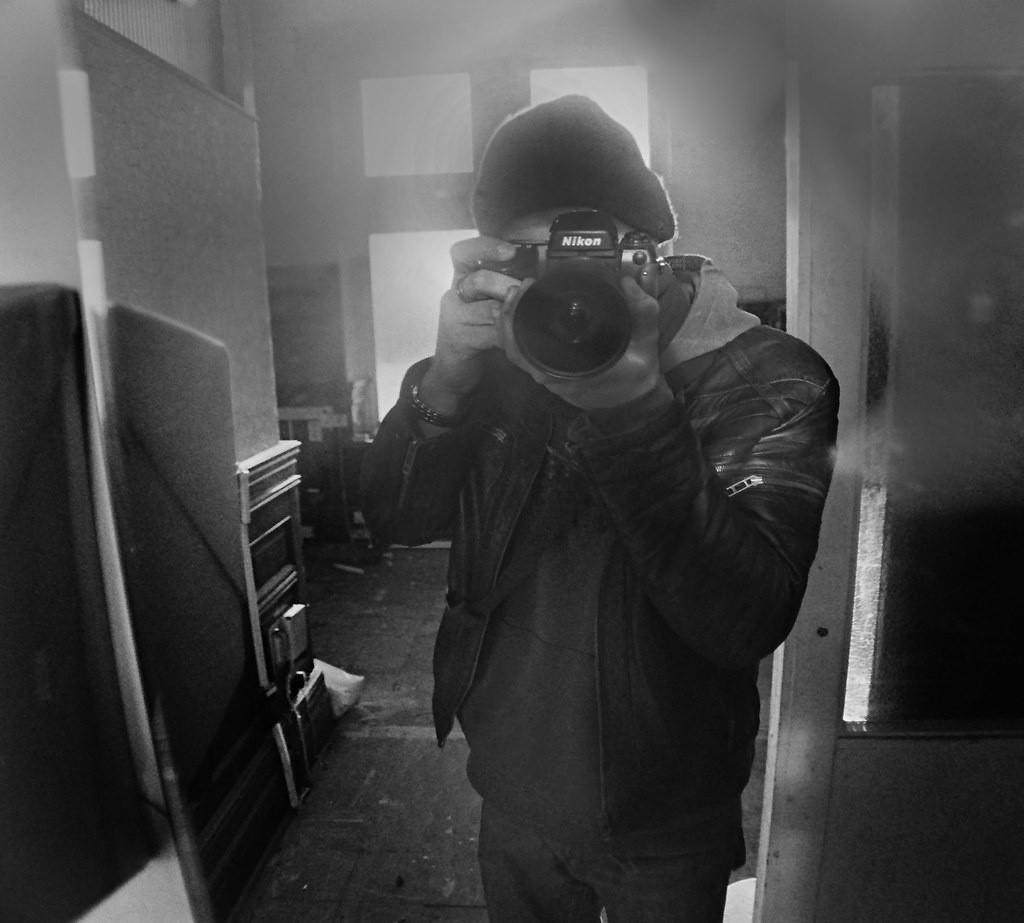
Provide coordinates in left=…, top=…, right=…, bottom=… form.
left=469, top=208, right=669, bottom=381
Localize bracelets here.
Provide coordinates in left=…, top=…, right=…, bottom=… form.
left=407, top=382, right=470, bottom=428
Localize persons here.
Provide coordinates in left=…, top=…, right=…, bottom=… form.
left=359, top=95, right=842, bottom=923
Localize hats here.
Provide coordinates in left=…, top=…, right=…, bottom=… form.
left=471, top=94, right=675, bottom=242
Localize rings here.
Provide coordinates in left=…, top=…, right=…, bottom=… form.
left=456, top=277, right=474, bottom=304
